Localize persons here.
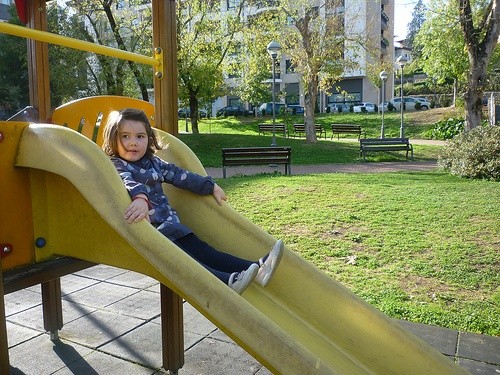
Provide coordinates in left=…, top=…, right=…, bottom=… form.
left=102, top=108, right=284, bottom=295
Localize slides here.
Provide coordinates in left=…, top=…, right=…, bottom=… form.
left=14, top=123, right=472, bottom=375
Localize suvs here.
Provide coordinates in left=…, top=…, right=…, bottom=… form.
left=260, top=102, right=286, bottom=115
left=389, top=97, right=430, bottom=111
left=325, top=103, right=351, bottom=114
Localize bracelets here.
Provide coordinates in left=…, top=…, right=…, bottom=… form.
left=131, top=196, right=151, bottom=207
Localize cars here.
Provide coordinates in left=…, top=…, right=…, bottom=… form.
left=378, top=102, right=388, bottom=113
left=216, top=106, right=254, bottom=117
left=353, top=101, right=377, bottom=113
left=179, top=107, right=210, bottom=120
left=287, top=105, right=304, bottom=116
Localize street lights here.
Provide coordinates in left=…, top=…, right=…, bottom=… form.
left=267, top=38, right=282, bottom=167
left=396, top=54, right=409, bottom=142
left=379, top=69, right=389, bottom=140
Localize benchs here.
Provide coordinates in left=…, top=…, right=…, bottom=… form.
left=258, top=124, right=290, bottom=138
left=221, top=146, right=292, bottom=177
left=293, top=124, right=327, bottom=140
left=360, top=137, right=414, bottom=162
left=331, top=123, right=367, bottom=141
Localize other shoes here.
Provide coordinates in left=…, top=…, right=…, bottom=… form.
left=252, top=240, right=284, bottom=288
left=229, top=263, right=259, bottom=296
